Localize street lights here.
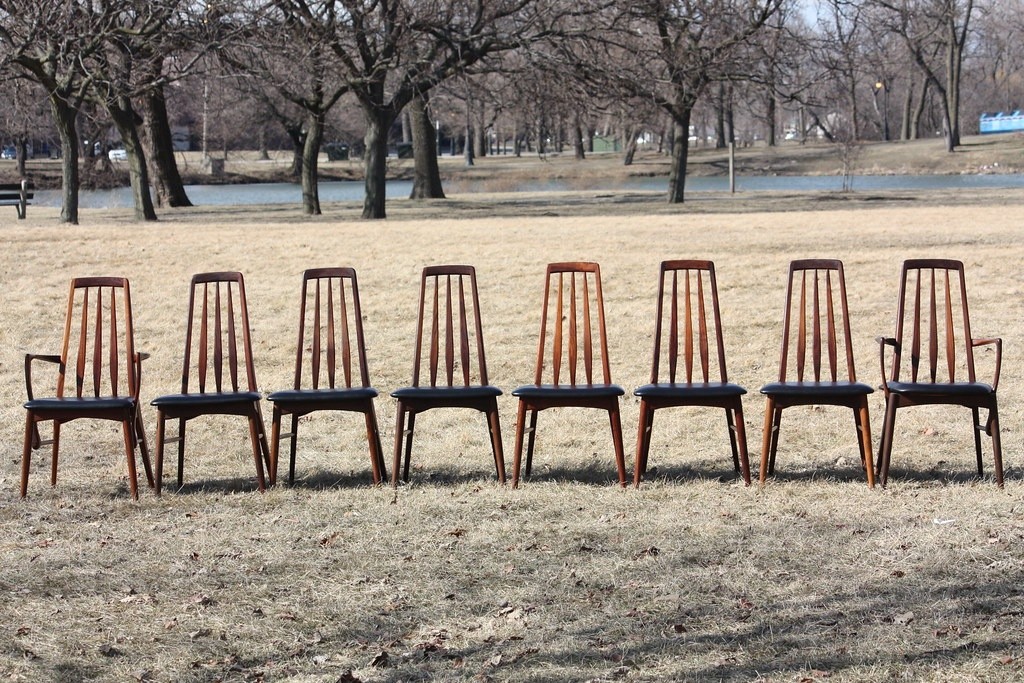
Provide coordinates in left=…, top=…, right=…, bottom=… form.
left=877, top=73, right=892, bottom=141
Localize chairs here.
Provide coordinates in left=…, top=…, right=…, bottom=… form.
left=390, top=265, right=506, bottom=490
left=759, top=259, right=874, bottom=490
left=633, top=260, right=751, bottom=489
left=266, top=267, right=388, bottom=488
left=20, top=276, right=155, bottom=501
left=512, top=261, right=628, bottom=492
left=150, top=271, right=273, bottom=498
left=876, top=259, right=1004, bottom=490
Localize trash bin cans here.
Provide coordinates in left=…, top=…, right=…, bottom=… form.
left=211, top=157, right=225, bottom=176
left=395, top=142, right=413, bottom=159
left=325, top=143, right=350, bottom=161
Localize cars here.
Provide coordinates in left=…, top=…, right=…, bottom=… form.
left=108, top=145, right=128, bottom=161
left=0, top=142, right=33, bottom=161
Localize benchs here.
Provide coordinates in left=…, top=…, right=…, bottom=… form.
left=0, top=180, right=34, bottom=219
